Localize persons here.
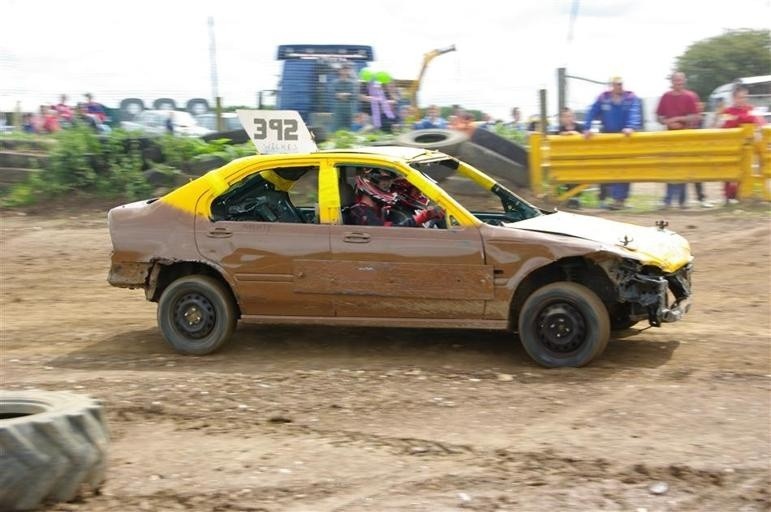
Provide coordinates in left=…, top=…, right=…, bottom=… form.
left=581, top=75, right=644, bottom=209
left=557, top=108, right=584, bottom=209
left=596, top=123, right=610, bottom=207
left=656, top=72, right=703, bottom=211
left=331, top=63, right=373, bottom=132
left=716, top=83, right=768, bottom=206
left=691, top=96, right=714, bottom=207
left=705, top=97, right=738, bottom=204
left=349, top=167, right=445, bottom=227
left=350, top=103, right=540, bottom=133
left=166, top=109, right=176, bottom=135
left=21, top=92, right=106, bottom=134
left=393, top=173, right=445, bottom=228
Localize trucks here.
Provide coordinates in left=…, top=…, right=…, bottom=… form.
left=703, top=73, right=771, bottom=129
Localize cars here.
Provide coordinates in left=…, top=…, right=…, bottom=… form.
left=545, top=120, right=604, bottom=134
left=107, top=143, right=697, bottom=367
left=1, top=108, right=244, bottom=139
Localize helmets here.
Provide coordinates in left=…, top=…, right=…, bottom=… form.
left=353, top=166, right=400, bottom=207
left=390, top=174, right=432, bottom=211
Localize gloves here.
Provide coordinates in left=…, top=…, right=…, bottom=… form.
left=411, top=205, right=447, bottom=225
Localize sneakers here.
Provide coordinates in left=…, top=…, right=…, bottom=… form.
left=557, top=197, right=743, bottom=210
